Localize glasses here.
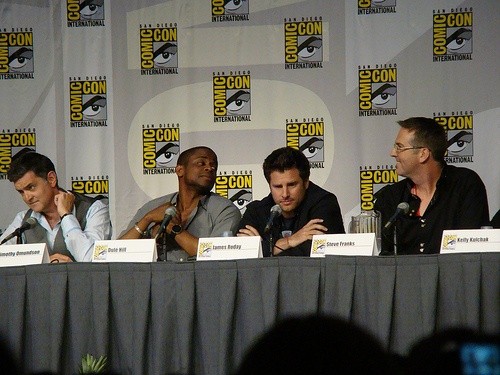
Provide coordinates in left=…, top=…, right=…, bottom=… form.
left=392, top=143, right=432, bottom=153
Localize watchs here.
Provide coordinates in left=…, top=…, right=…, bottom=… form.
left=170, top=224, right=184, bottom=240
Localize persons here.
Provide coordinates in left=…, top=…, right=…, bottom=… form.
left=0, top=152, right=113, bottom=263
left=234, top=146, right=345, bottom=256
left=236, top=312, right=500, bottom=375
left=118, top=146, right=241, bottom=262
left=373, top=117, right=489, bottom=254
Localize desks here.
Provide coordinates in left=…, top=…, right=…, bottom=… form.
left=0, top=253, right=500, bottom=375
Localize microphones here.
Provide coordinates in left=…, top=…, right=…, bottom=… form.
left=264, top=204, right=282, bottom=232
left=155, top=206, right=177, bottom=239
left=1, top=217, right=38, bottom=245
left=385, top=202, right=410, bottom=230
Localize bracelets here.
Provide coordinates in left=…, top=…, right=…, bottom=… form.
left=135, top=223, right=143, bottom=234
left=286, top=238, right=290, bottom=248
left=61, top=213, right=73, bottom=220
